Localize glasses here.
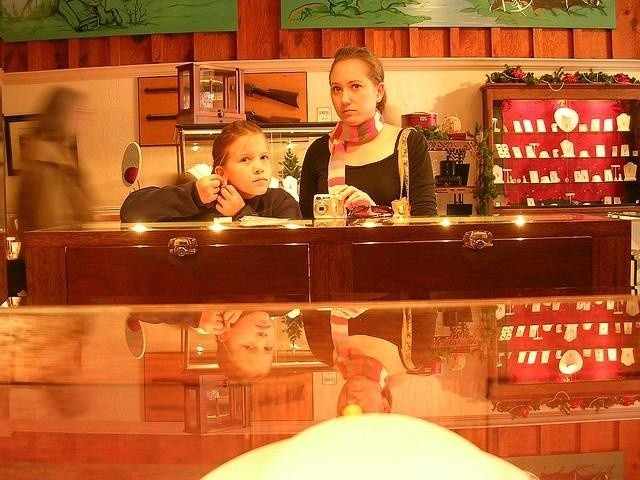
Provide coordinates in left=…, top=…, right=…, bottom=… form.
left=348, top=205, right=395, bottom=218
left=346, top=218, right=394, bottom=225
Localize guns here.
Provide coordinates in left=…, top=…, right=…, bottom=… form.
left=144, top=82, right=300, bottom=108
left=146, top=111, right=300, bottom=124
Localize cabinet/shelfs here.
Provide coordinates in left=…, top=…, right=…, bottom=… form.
left=486, top=296, right=640, bottom=385
left=176, top=63, right=246, bottom=126
left=183, top=377, right=249, bottom=437
left=427, top=139, right=482, bottom=217
left=482, top=82, right=640, bottom=213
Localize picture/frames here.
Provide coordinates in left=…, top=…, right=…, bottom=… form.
left=0, top=313, right=90, bottom=385
left=4, top=113, right=78, bottom=177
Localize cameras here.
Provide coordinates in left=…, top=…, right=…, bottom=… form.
left=313, top=193, right=348, bottom=219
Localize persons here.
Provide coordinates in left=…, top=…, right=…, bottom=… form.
left=300, top=45, right=440, bottom=218
left=125, top=310, right=297, bottom=383
left=16, top=86, right=101, bottom=229
left=121, top=120, right=302, bottom=221
left=301, top=309, right=439, bottom=423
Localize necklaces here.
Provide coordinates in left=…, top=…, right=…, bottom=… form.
left=494, top=168, right=501, bottom=177
left=551, top=115, right=635, bottom=182
